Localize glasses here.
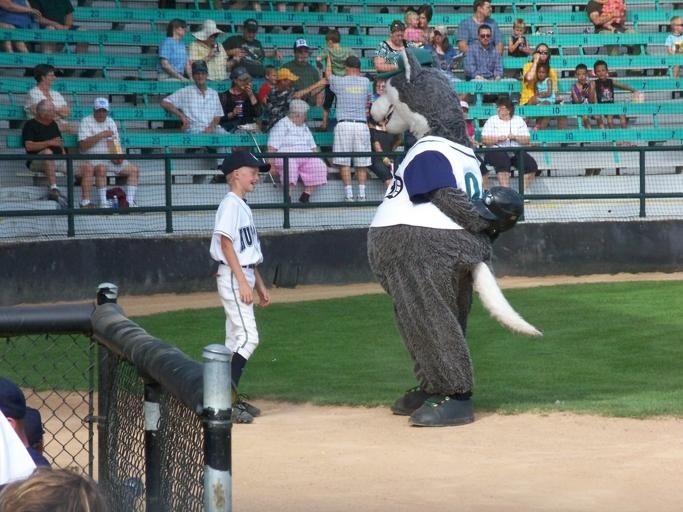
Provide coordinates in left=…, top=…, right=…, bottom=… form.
left=481, top=34, right=490, bottom=37
left=538, top=51, right=548, bottom=54
left=211, top=34, right=217, bottom=38
left=674, top=23, right=682, bottom=26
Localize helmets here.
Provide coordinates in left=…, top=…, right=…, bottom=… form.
left=473, top=187, right=524, bottom=220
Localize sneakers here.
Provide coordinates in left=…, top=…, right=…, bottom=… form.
left=411, top=396, right=474, bottom=426
left=128, top=199, right=138, bottom=207
left=300, top=191, right=310, bottom=203
left=49, top=188, right=60, bottom=198
left=230, top=402, right=253, bottom=423
left=78, top=202, right=95, bottom=209
left=236, top=400, right=259, bottom=416
left=357, top=194, right=367, bottom=201
left=344, top=195, right=354, bottom=201
left=390, top=385, right=433, bottom=414
left=513, top=74, right=523, bottom=81
left=99, top=201, right=111, bottom=207
left=533, top=124, right=541, bottom=131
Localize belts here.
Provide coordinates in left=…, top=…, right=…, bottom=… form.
left=340, top=119, right=366, bottom=123
left=220, top=261, right=255, bottom=269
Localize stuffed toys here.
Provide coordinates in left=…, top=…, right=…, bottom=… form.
left=363, top=39, right=543, bottom=427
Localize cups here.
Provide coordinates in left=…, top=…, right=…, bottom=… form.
left=234, top=100, right=245, bottom=117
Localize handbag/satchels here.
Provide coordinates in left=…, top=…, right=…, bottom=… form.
left=240, top=45, right=264, bottom=66
left=106, top=188, right=128, bottom=208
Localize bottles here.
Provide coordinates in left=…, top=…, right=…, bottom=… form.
left=112, top=195, right=119, bottom=216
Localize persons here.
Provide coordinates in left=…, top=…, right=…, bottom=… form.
left=1, top=0, right=682, bottom=208
left=0, top=375, right=112, bottom=512
left=208, top=149, right=271, bottom=425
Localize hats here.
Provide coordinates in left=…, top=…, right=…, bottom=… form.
left=231, top=67, right=250, bottom=80
left=171, top=19, right=190, bottom=32
left=295, top=39, right=308, bottom=50
left=343, top=56, right=360, bottom=67
left=0, top=378, right=25, bottom=418
left=245, top=19, right=257, bottom=30
left=93, top=98, right=109, bottom=110
left=434, top=25, right=447, bottom=35
left=278, top=68, right=299, bottom=80
left=461, top=100, right=468, bottom=108
left=288, top=99, right=310, bottom=113
left=192, top=61, right=208, bottom=72
left=193, top=20, right=224, bottom=41
left=25, top=407, right=45, bottom=446
left=221, top=152, right=271, bottom=175
